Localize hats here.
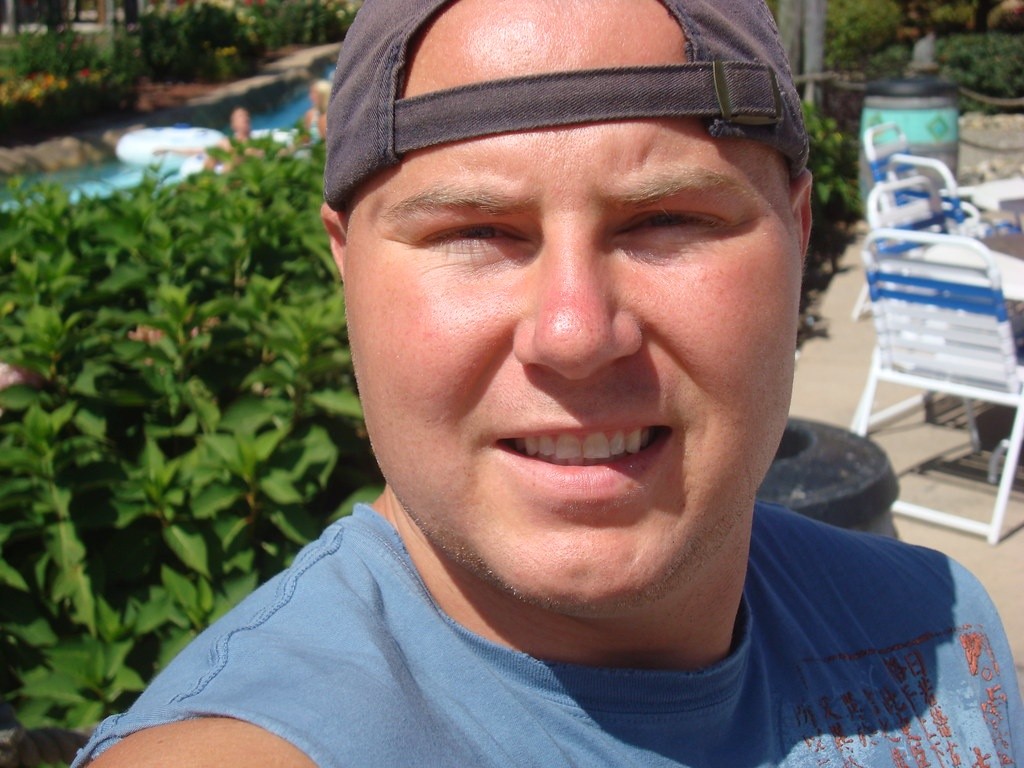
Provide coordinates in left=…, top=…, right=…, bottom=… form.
left=323, top=0, right=807, bottom=211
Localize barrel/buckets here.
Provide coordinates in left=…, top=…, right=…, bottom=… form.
left=860, top=68, right=959, bottom=216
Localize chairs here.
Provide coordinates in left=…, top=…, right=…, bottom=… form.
left=851, top=228, right=1024, bottom=549
left=866, top=174, right=1024, bottom=302
left=849, top=154, right=988, bottom=320
left=865, top=121, right=918, bottom=186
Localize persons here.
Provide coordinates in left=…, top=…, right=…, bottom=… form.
left=290, top=76, right=332, bottom=150
left=62, top=0, right=1024, bottom=768
left=201, top=109, right=272, bottom=176
